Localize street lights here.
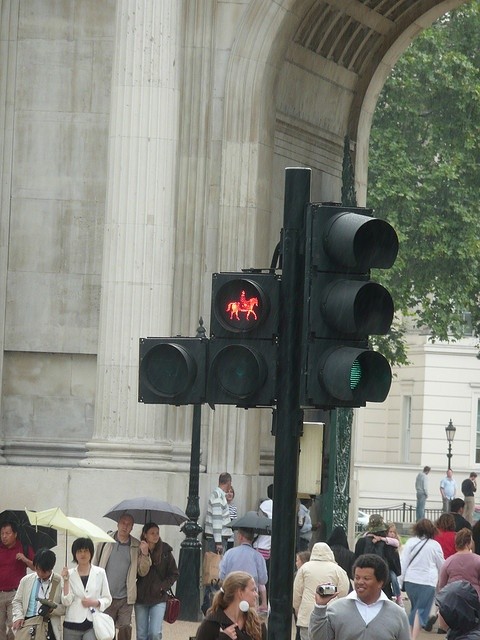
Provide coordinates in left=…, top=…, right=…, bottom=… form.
left=445, top=419, right=456, bottom=469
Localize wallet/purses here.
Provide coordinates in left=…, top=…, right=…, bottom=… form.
left=40, top=599, right=57, bottom=609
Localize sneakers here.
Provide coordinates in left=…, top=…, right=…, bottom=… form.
left=425, top=615, right=437, bottom=631
left=395, top=600, right=405, bottom=612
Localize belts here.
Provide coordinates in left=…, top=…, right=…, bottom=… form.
left=0, top=587, right=18, bottom=592
left=12, top=574, right=61, bottom=640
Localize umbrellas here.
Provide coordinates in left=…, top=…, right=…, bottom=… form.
left=22, top=506, right=118, bottom=581
left=103, top=498, right=191, bottom=539
left=219, top=512, right=273, bottom=536
left=1, top=509, right=57, bottom=551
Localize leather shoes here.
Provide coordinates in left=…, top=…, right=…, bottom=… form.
left=437, top=625, right=450, bottom=634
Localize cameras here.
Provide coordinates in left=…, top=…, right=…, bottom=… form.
left=316, top=585, right=337, bottom=596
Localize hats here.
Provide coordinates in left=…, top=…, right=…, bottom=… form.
left=369, top=514, right=391, bottom=532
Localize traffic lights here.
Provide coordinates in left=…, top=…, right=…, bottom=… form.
left=140, top=339, right=205, bottom=403
left=210, top=273, right=276, bottom=404
left=305, top=206, right=399, bottom=408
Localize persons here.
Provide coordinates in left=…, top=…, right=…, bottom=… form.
left=134, top=523, right=180, bottom=639
left=439, top=470, right=456, bottom=514
left=432, top=514, right=458, bottom=559
left=292, top=540, right=350, bottom=639
left=223, top=485, right=236, bottom=553
left=353, top=513, right=402, bottom=576
left=414, top=465, right=430, bottom=523
left=296, top=548, right=309, bottom=575
left=253, top=483, right=273, bottom=575
left=61, top=537, right=112, bottom=640
left=367, top=522, right=401, bottom=547
left=460, top=472, right=476, bottom=525
left=437, top=528, right=480, bottom=604
left=11, top=548, right=67, bottom=639
left=308, top=554, right=412, bottom=640
left=204, top=473, right=233, bottom=584
left=449, top=499, right=472, bottom=532
left=1, top=521, right=35, bottom=640
left=362, top=522, right=404, bottom=608
left=195, top=571, right=264, bottom=640
left=400, top=518, right=445, bottom=639
left=324, top=525, right=357, bottom=581
left=296, top=496, right=312, bottom=553
left=434, top=580, right=479, bottom=640
left=218, top=527, right=267, bottom=614
left=91, top=515, right=152, bottom=638
left=471, top=519, right=479, bottom=555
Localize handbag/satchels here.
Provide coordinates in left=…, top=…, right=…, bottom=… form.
left=201, top=578, right=220, bottom=616
left=90, top=609, right=116, bottom=640
left=164, top=591, right=181, bottom=624
left=202, top=549, right=224, bottom=584
left=257, top=548, right=270, bottom=560
left=188, top=623, right=226, bottom=640
left=401, top=538, right=429, bottom=592
